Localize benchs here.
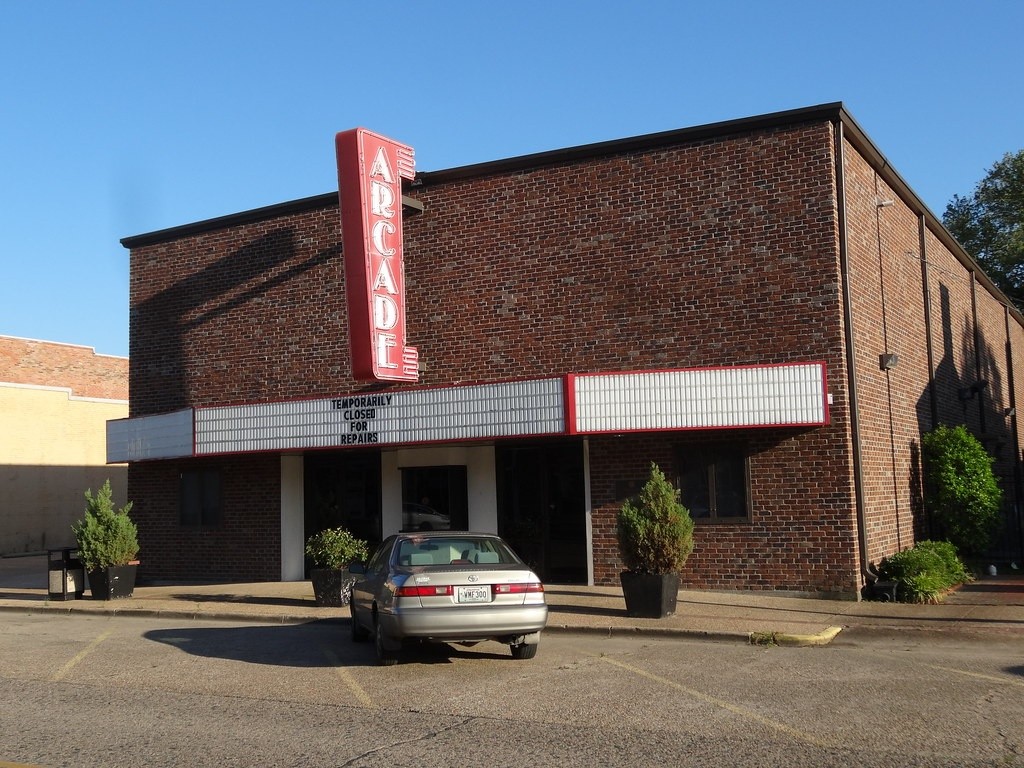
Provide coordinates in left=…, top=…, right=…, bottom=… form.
left=408, top=552, right=499, bottom=564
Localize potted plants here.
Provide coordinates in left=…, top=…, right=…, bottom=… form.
left=615, top=461, right=696, bottom=619
left=302, top=526, right=369, bottom=608
left=70, top=477, right=140, bottom=601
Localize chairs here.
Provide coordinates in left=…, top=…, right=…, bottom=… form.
left=400, top=555, right=408, bottom=565
left=461, top=549, right=481, bottom=563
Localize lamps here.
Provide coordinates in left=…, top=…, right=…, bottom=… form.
left=883, top=354, right=898, bottom=369
left=1005, top=408, right=1014, bottom=416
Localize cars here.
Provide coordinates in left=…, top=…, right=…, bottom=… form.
left=349, top=529, right=547, bottom=665
left=405, top=503, right=457, bottom=530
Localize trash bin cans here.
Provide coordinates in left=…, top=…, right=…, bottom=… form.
left=45, top=537, right=86, bottom=601
